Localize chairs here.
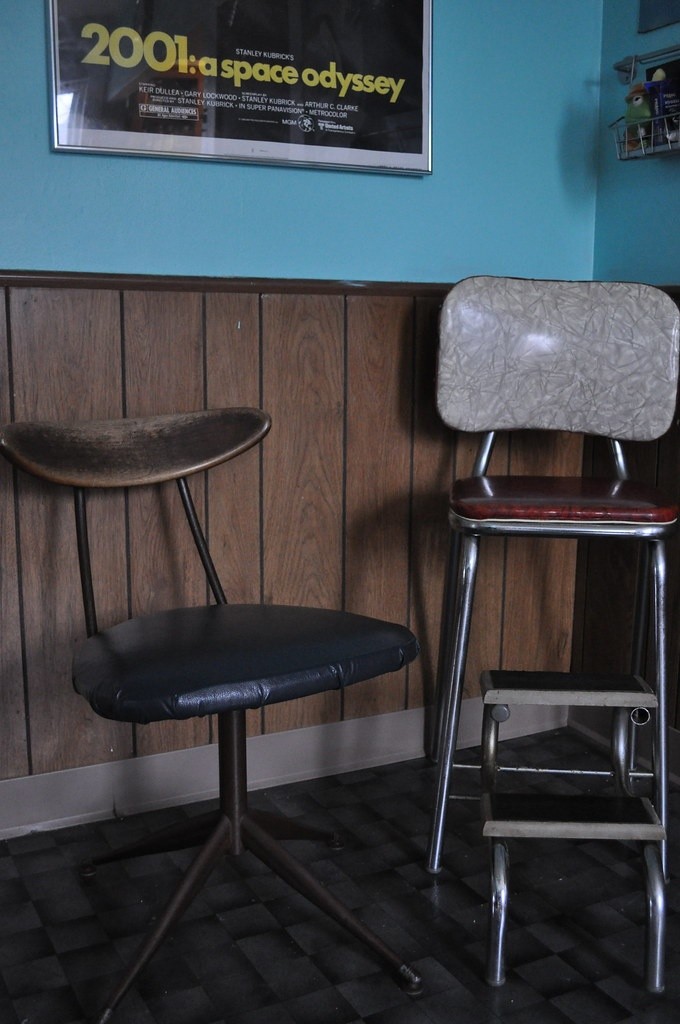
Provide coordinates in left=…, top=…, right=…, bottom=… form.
left=0, top=408, right=425, bottom=1024
left=427, top=275, right=680, bottom=884
left=477, top=671, right=668, bottom=994
